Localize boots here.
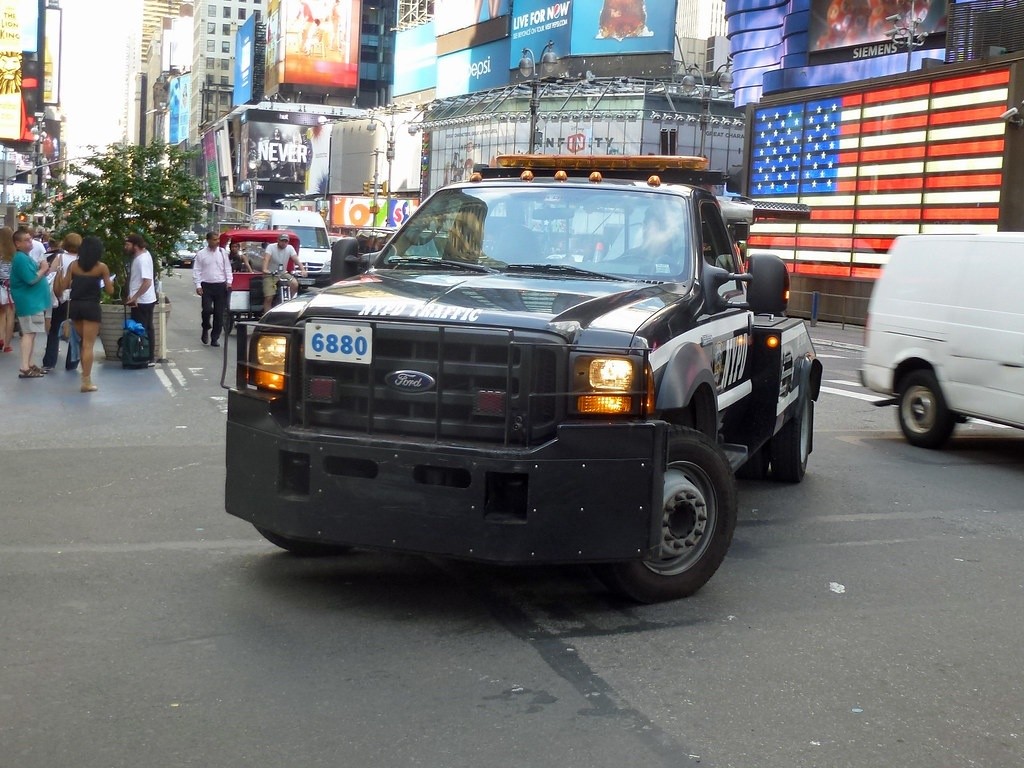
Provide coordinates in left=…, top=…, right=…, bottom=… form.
left=81, top=374, right=98, bottom=391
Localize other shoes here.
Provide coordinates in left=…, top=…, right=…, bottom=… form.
left=211, top=341, right=220, bottom=346
left=0, top=339, right=4, bottom=350
left=201, top=329, right=208, bottom=345
left=42, top=366, right=54, bottom=371
left=4, top=347, right=13, bottom=352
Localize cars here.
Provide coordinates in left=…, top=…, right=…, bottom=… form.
left=162, top=242, right=196, bottom=268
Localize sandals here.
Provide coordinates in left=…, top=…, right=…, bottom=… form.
left=29, top=364, right=47, bottom=374
left=19, top=367, right=44, bottom=378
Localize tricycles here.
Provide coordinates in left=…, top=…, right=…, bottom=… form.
left=213, top=231, right=302, bottom=335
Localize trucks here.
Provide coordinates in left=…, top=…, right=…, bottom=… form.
left=245, top=208, right=331, bottom=290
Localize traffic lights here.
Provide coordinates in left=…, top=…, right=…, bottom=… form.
left=20, top=59, right=39, bottom=115
left=19, top=213, right=26, bottom=221
left=363, top=179, right=370, bottom=197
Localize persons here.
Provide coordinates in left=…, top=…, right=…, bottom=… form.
left=192, top=231, right=233, bottom=347
left=262, top=233, right=308, bottom=314
left=124, top=231, right=158, bottom=367
left=292, top=0, right=340, bottom=57
left=227, top=242, right=252, bottom=273
left=621, top=205, right=684, bottom=271
left=444, top=206, right=500, bottom=264
left=0, top=225, right=113, bottom=393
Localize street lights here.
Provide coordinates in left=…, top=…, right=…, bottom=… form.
left=518, top=40, right=562, bottom=155
left=679, top=55, right=734, bottom=158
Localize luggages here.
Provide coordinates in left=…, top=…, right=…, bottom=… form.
left=117, top=303, right=150, bottom=369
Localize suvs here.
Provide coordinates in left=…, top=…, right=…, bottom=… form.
left=224, top=154, right=818, bottom=601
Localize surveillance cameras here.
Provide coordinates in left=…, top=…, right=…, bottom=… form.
left=917, top=32, right=928, bottom=41
left=1000, top=108, right=1018, bottom=120
left=914, top=18, right=922, bottom=27
left=886, top=14, right=901, bottom=22
left=35, top=112, right=45, bottom=118
left=883, top=29, right=900, bottom=37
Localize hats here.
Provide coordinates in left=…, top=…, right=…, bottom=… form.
left=280, top=234, right=289, bottom=242
left=126, top=234, right=149, bottom=248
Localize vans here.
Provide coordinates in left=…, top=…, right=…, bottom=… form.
left=856, top=231, right=1024, bottom=450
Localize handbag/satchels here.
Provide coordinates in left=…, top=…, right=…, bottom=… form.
left=63, top=321, right=71, bottom=338
left=53, top=254, right=64, bottom=297
